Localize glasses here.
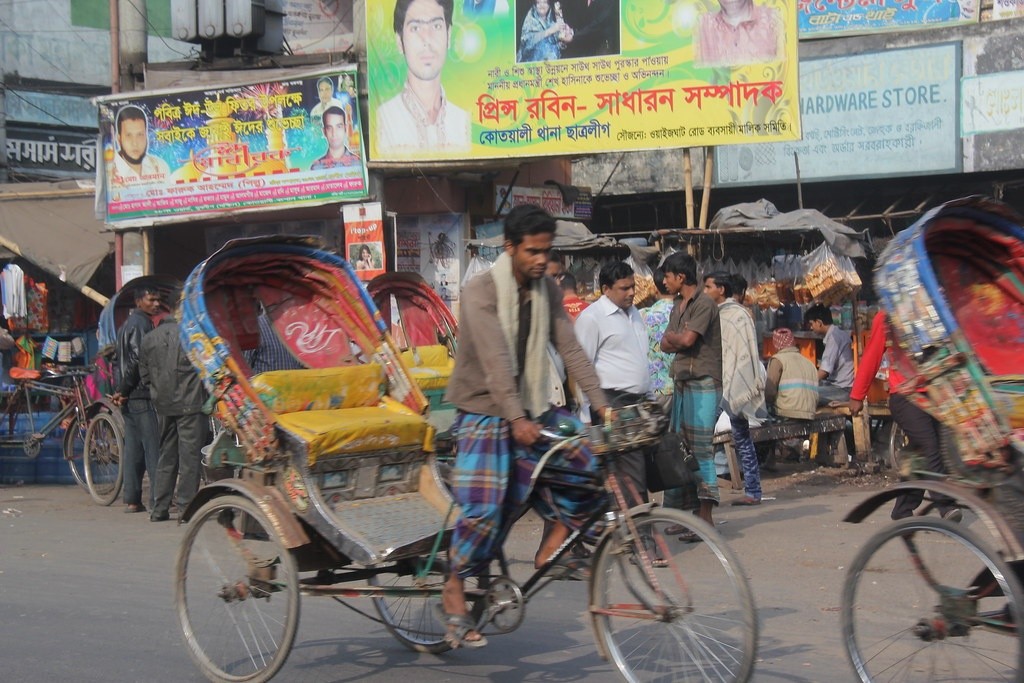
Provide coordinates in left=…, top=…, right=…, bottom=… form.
left=549, top=272, right=565, bottom=282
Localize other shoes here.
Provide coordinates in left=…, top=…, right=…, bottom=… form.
left=628, top=547, right=668, bottom=568
left=125, top=503, right=147, bottom=513
left=664, top=523, right=690, bottom=535
left=940, top=505, right=963, bottom=523
left=150, top=516, right=169, bottom=522
left=732, top=496, right=760, bottom=506
left=679, top=531, right=704, bottom=542
left=568, top=537, right=591, bottom=558
left=177, top=517, right=189, bottom=525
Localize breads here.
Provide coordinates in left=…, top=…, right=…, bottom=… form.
left=631, top=272, right=659, bottom=308
left=738, top=258, right=863, bottom=308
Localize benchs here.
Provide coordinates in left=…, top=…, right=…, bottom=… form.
left=710, top=409, right=851, bottom=484
left=256, top=361, right=429, bottom=457
left=839, top=406, right=892, bottom=454
left=393, top=347, right=462, bottom=398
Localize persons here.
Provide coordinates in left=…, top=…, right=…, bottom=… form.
left=310, top=106, right=363, bottom=171
left=112, top=105, right=168, bottom=187
left=517, top=0, right=619, bottom=63
left=539, top=251, right=854, bottom=569
left=311, top=76, right=356, bottom=128
left=109, top=286, right=303, bottom=539
left=356, top=244, right=373, bottom=271
left=694, top=0, right=785, bottom=68
left=442, top=202, right=620, bottom=651
left=376, top=0, right=471, bottom=152
left=851, top=310, right=963, bottom=524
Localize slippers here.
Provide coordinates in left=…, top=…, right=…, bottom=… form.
left=431, top=602, right=489, bottom=648
left=540, top=555, right=595, bottom=581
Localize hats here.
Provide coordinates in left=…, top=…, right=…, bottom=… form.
left=772, top=327, right=794, bottom=350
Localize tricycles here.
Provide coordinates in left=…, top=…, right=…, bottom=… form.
left=173, top=232, right=760, bottom=683
left=365, top=269, right=458, bottom=436
left=839, top=194, right=1024, bottom=683
left=0, top=327, right=126, bottom=506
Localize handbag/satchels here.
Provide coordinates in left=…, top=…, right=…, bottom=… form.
left=644, top=431, right=701, bottom=494
